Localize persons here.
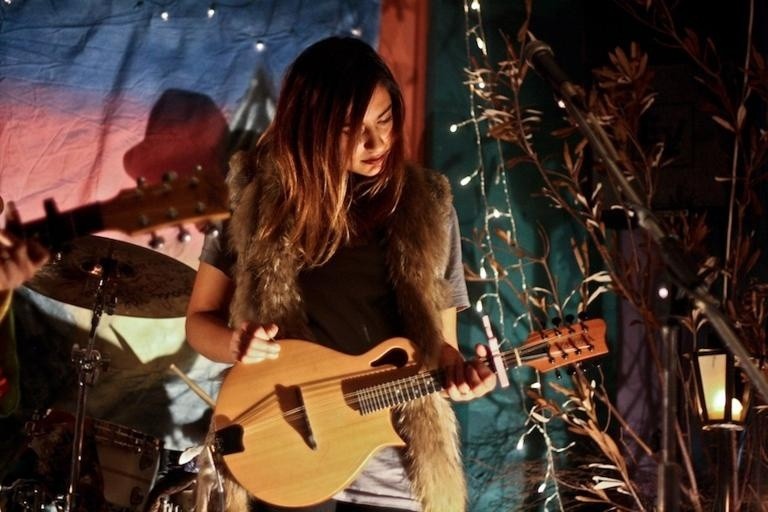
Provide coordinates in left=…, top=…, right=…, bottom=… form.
left=0, top=196, right=54, bottom=320
left=179, top=29, right=499, bottom=511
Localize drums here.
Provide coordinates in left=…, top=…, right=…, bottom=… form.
left=17, top=408, right=164, bottom=511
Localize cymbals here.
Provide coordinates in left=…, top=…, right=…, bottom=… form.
left=19, top=236, right=198, bottom=318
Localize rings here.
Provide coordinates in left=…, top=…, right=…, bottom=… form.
left=460, top=389, right=473, bottom=396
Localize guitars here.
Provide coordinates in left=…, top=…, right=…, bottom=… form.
left=1, top=166, right=231, bottom=331
left=213, top=319, right=609, bottom=508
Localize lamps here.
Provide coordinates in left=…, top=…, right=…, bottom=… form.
left=691, top=348, right=763, bottom=431
